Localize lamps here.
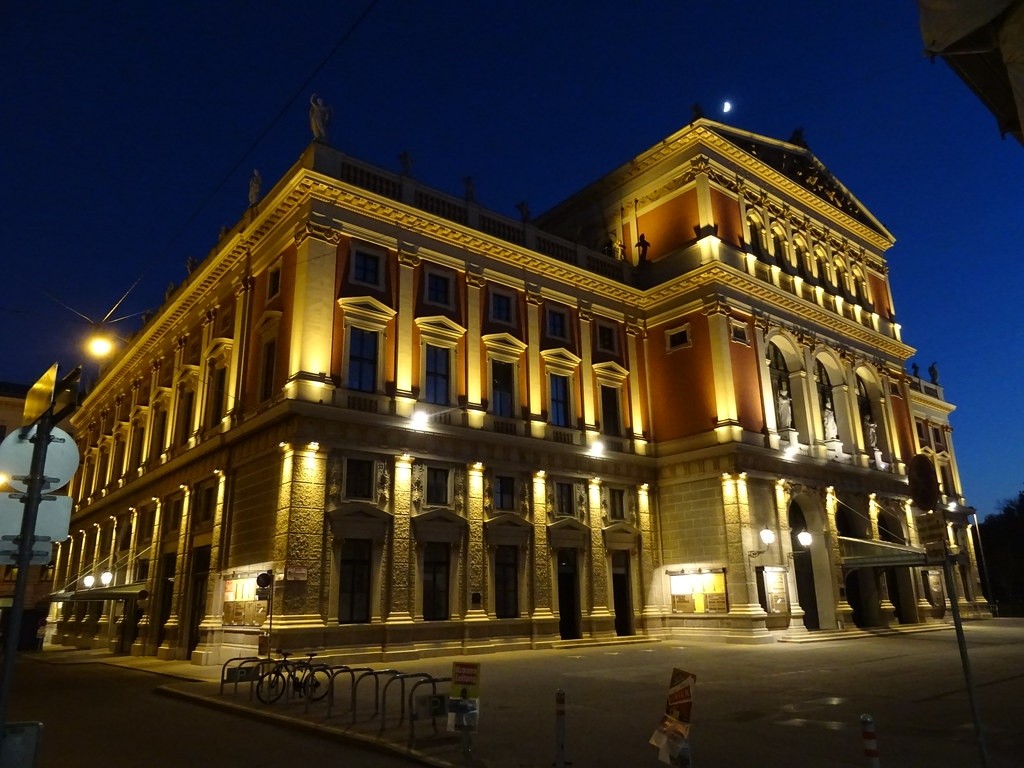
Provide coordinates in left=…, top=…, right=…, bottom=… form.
left=83, top=575, right=95, bottom=588
left=748, top=524, right=775, bottom=558
left=101, top=571, right=113, bottom=586
left=788, top=527, right=813, bottom=559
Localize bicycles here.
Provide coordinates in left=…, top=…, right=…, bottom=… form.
left=255, top=651, right=333, bottom=704
